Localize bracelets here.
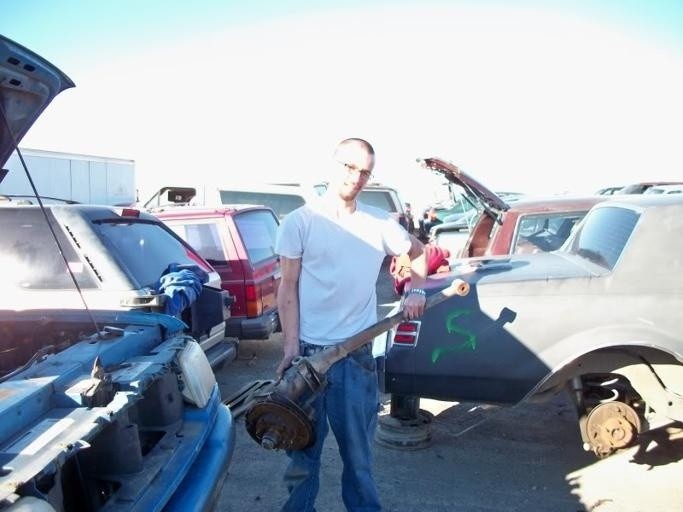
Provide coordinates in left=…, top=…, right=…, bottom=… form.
left=405, top=288, right=426, bottom=298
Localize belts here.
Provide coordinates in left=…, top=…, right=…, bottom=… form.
left=307, top=345, right=372, bottom=357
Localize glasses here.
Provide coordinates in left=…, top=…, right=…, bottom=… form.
left=337, top=163, right=371, bottom=177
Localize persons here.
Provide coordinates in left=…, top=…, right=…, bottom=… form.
left=274, top=138, right=428, bottom=512
